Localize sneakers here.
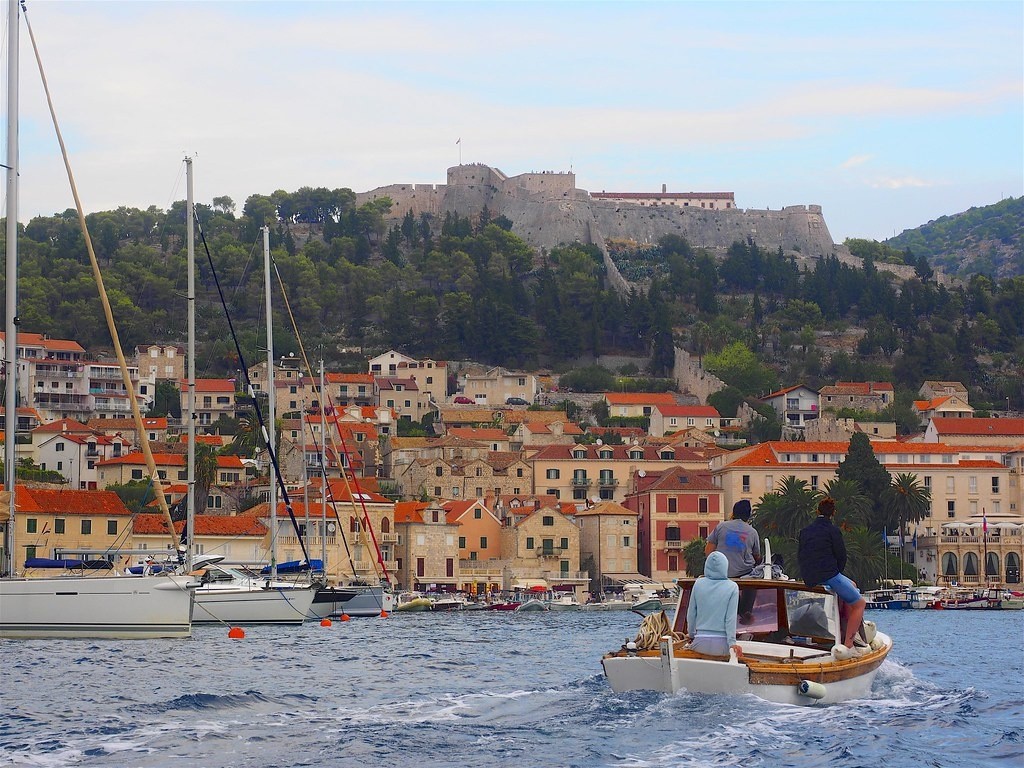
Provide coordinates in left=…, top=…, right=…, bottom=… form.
left=850, top=645, right=862, bottom=657
left=854, top=632, right=866, bottom=647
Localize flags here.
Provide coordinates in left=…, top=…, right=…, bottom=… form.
left=912, top=530, right=917, bottom=548
left=898, top=527, right=904, bottom=548
left=162, top=494, right=188, bottom=527
left=882, top=530, right=889, bottom=547
left=179, top=522, right=187, bottom=554
left=983, top=517, right=988, bottom=534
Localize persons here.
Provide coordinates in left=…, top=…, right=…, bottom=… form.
left=754, top=554, right=785, bottom=612
left=844, top=600, right=877, bottom=650
left=704, top=500, right=762, bottom=625
left=797, top=497, right=866, bottom=658
left=685, top=550, right=743, bottom=656
left=948, top=528, right=969, bottom=536
left=360, top=578, right=670, bottom=605
left=143, top=558, right=152, bottom=577
left=919, top=567, right=928, bottom=581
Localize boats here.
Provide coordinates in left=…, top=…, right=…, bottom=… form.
left=245, top=223, right=396, bottom=615
left=599, top=537, right=893, bottom=704
left=395, top=581, right=1024, bottom=611
left=0, top=0, right=197, bottom=640
left=124, top=155, right=321, bottom=625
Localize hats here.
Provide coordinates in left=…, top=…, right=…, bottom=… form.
left=733, top=500, right=751, bottom=519
